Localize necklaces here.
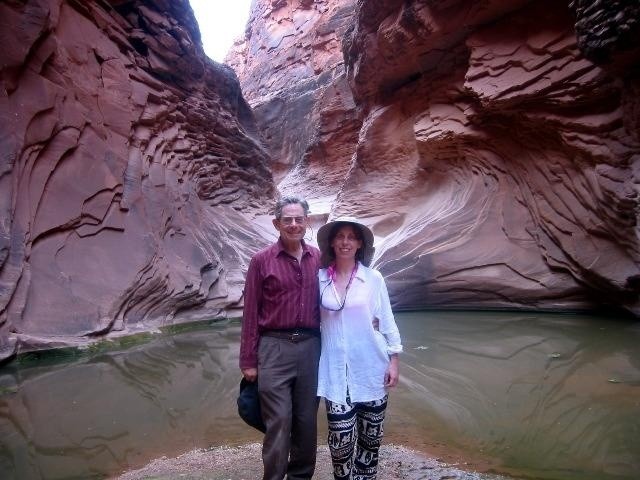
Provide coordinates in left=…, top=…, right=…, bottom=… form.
left=319, top=260, right=356, bottom=313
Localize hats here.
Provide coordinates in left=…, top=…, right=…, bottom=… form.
left=238, top=376, right=266, bottom=434
left=316, top=217, right=374, bottom=253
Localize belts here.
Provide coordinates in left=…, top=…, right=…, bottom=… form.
left=264, top=331, right=316, bottom=341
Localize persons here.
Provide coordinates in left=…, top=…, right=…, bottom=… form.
left=312, top=214, right=406, bottom=480
left=238, top=195, right=381, bottom=480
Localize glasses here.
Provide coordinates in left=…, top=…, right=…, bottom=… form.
left=334, top=234, right=356, bottom=240
